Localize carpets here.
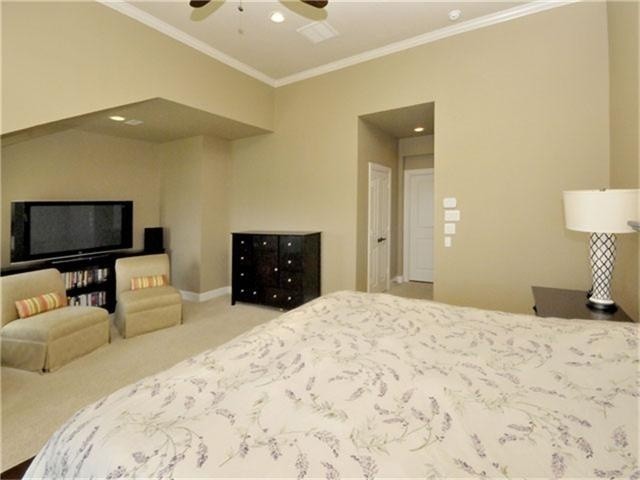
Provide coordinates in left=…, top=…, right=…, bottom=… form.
left=1, top=456, right=34, bottom=479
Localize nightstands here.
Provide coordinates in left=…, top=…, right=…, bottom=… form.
left=529, top=287, right=635, bottom=320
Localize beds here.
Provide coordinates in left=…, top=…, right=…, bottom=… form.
left=19, top=287, right=640, bottom=480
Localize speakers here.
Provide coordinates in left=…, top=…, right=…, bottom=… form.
left=144, top=227, right=164, bottom=251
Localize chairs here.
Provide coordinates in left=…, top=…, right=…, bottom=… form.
left=115, top=253, right=185, bottom=341
left=0, top=268, right=111, bottom=373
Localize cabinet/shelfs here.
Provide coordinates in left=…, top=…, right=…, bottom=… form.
left=0, top=250, right=138, bottom=313
left=231, top=230, right=321, bottom=309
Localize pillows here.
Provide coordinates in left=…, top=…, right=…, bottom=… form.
left=13, top=292, right=69, bottom=318
left=125, top=274, right=169, bottom=291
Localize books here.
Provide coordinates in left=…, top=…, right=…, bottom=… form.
left=62, top=266, right=109, bottom=308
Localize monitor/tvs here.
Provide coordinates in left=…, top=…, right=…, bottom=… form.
left=10, top=201, right=134, bottom=265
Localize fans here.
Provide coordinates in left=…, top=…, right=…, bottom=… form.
left=190, top=0, right=329, bottom=11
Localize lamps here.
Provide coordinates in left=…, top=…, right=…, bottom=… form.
left=562, top=184, right=640, bottom=310
